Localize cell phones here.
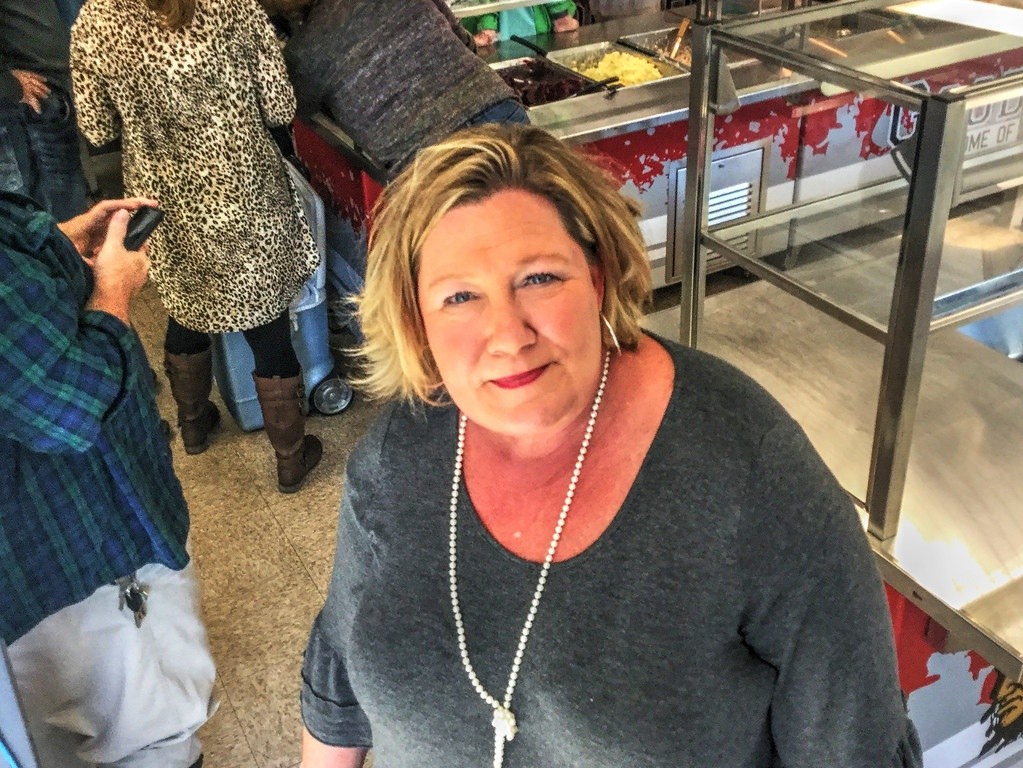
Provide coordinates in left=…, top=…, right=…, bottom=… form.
left=122, top=206, right=164, bottom=252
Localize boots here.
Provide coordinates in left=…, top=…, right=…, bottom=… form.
left=250, top=365, right=324, bottom=492
left=161, top=344, right=223, bottom=455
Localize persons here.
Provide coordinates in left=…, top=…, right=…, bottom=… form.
left=1, top=0, right=172, bottom=444
left=261, top=0, right=535, bottom=194
left=459, top=0, right=581, bottom=48
left=69, top=0, right=324, bottom=494
left=0, top=188, right=222, bottom=767
left=298, top=124, right=922, bottom=768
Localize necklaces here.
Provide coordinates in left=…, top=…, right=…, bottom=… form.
left=447, top=346, right=612, bottom=767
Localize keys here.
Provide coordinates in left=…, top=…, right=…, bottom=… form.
left=112, top=570, right=151, bottom=629
